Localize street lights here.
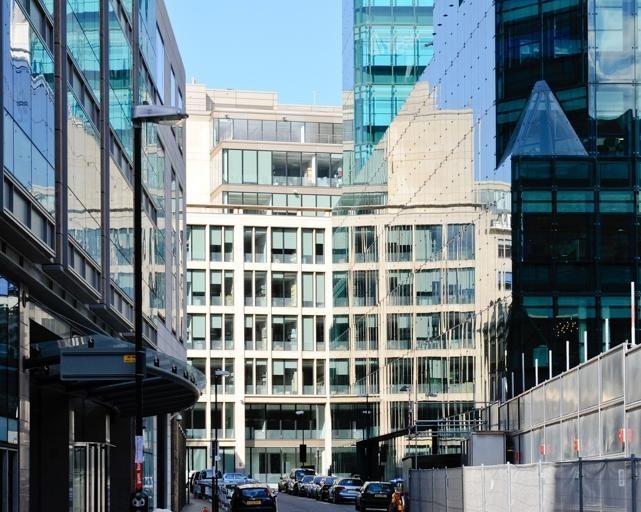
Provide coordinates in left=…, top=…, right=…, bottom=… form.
left=295, top=409, right=305, bottom=467
left=214, top=369, right=230, bottom=511
left=400, top=384, right=412, bottom=456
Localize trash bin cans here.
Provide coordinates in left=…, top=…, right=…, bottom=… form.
left=130, top=492, right=148, bottom=512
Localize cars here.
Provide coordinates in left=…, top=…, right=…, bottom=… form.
left=279, top=467, right=337, bottom=500
left=143, top=476, right=154, bottom=508
left=356, top=481, right=396, bottom=510
left=186, top=470, right=278, bottom=512
left=330, top=477, right=364, bottom=503
